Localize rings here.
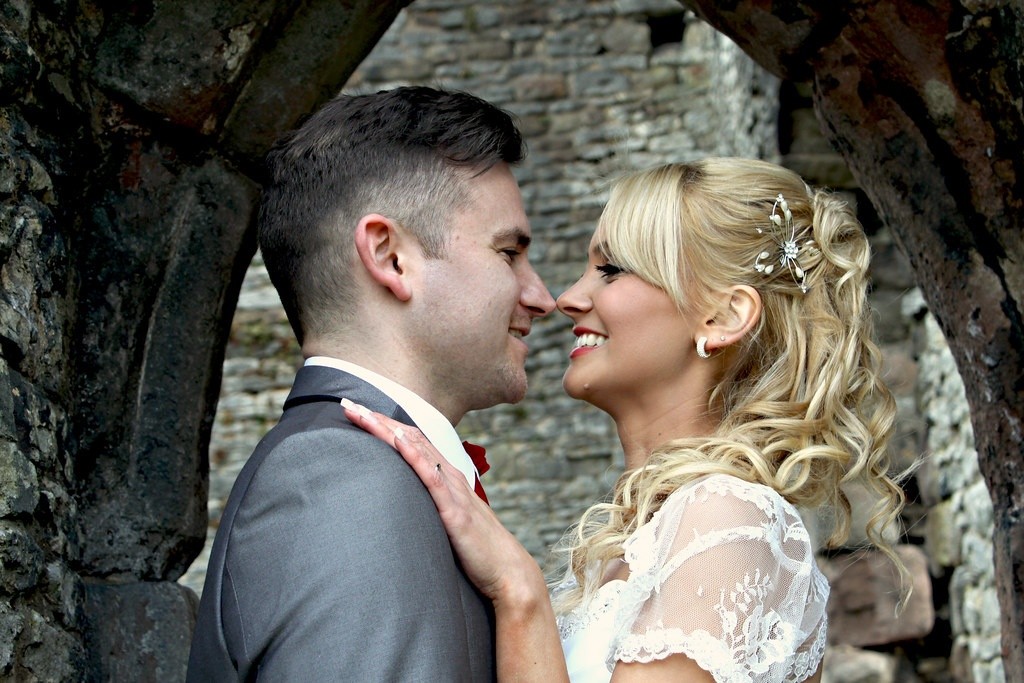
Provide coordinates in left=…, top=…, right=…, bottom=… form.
left=435, top=464, right=441, bottom=470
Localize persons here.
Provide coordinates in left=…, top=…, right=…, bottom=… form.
left=187, top=78, right=557, bottom=683
left=339, top=153, right=930, bottom=683
